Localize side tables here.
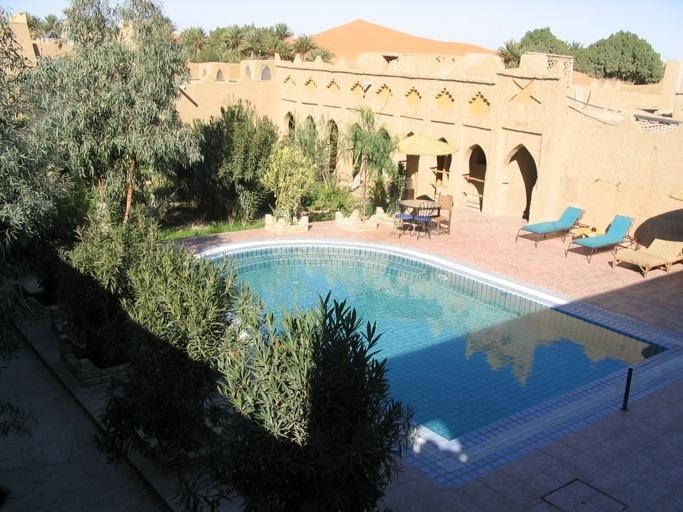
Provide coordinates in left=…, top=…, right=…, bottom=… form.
left=616, top=240, right=646, bottom=250
left=569, top=227, right=604, bottom=237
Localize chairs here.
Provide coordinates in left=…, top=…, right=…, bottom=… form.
left=611, top=238, right=683, bottom=279
left=565, top=214, right=633, bottom=263
left=263, top=194, right=453, bottom=241
left=515, top=207, right=585, bottom=248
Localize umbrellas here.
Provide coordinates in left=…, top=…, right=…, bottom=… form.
left=391, top=131, right=458, bottom=201
